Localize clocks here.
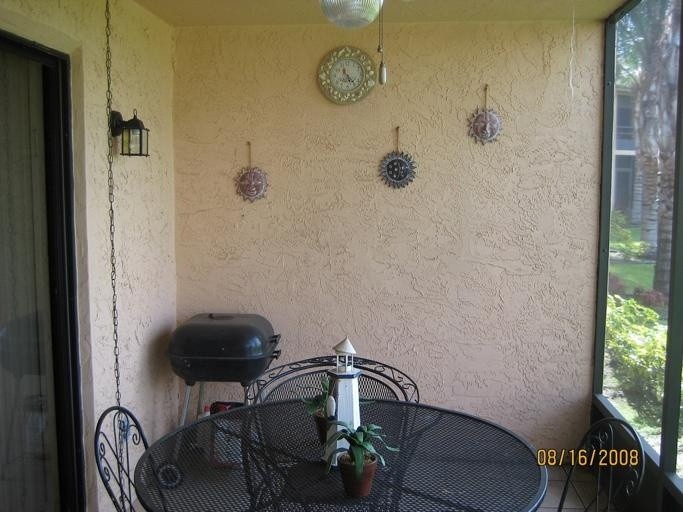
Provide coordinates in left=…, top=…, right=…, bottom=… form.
left=316, top=47, right=376, bottom=104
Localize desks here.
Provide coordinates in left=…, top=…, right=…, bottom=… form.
left=134, top=394, right=550, bottom=511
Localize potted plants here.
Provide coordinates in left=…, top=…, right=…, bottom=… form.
left=319, top=419, right=401, bottom=496
left=291, top=374, right=328, bottom=446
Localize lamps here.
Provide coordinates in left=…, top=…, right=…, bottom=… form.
left=110, top=109, right=150, bottom=158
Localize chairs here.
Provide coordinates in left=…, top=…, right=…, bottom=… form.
left=92, top=405, right=168, bottom=512
left=556, top=415, right=646, bottom=511
left=241, top=353, right=419, bottom=511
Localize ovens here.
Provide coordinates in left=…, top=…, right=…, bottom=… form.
left=164, top=311, right=282, bottom=387
